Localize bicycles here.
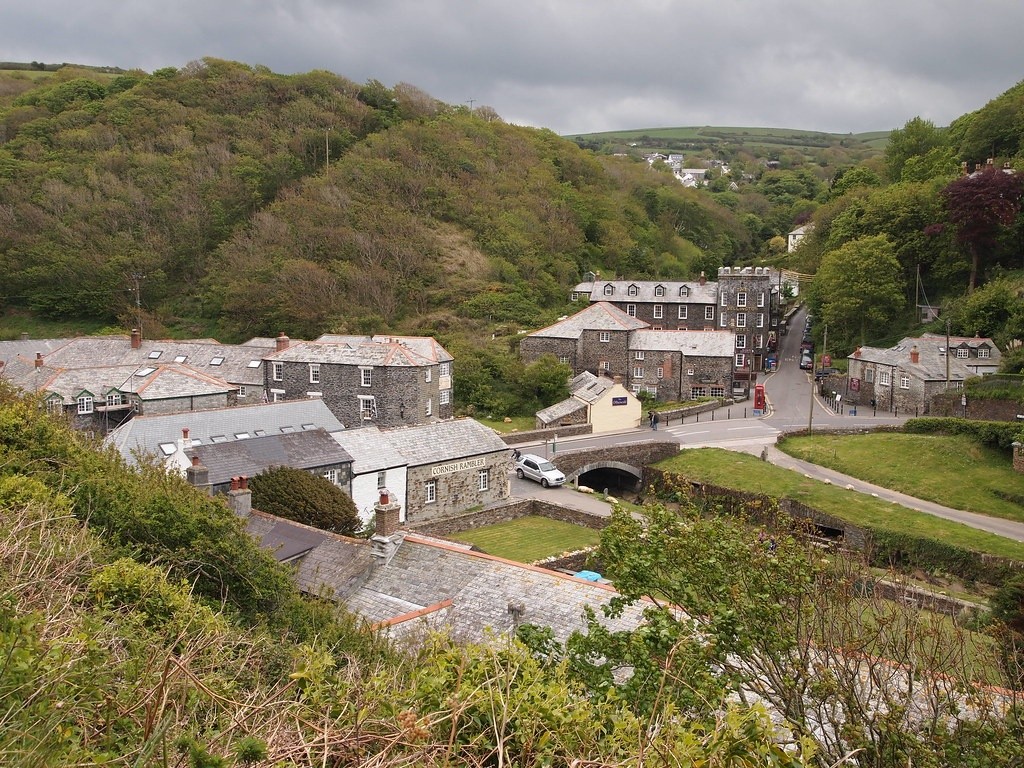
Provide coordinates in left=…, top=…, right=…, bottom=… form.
left=507, top=457, right=516, bottom=471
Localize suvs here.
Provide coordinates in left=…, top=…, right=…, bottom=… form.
left=514, top=454, right=566, bottom=488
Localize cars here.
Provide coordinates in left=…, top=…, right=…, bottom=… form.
left=799, top=314, right=814, bottom=370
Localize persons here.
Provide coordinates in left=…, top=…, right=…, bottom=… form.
left=647, top=410, right=659, bottom=431
left=511, top=449, right=521, bottom=461
